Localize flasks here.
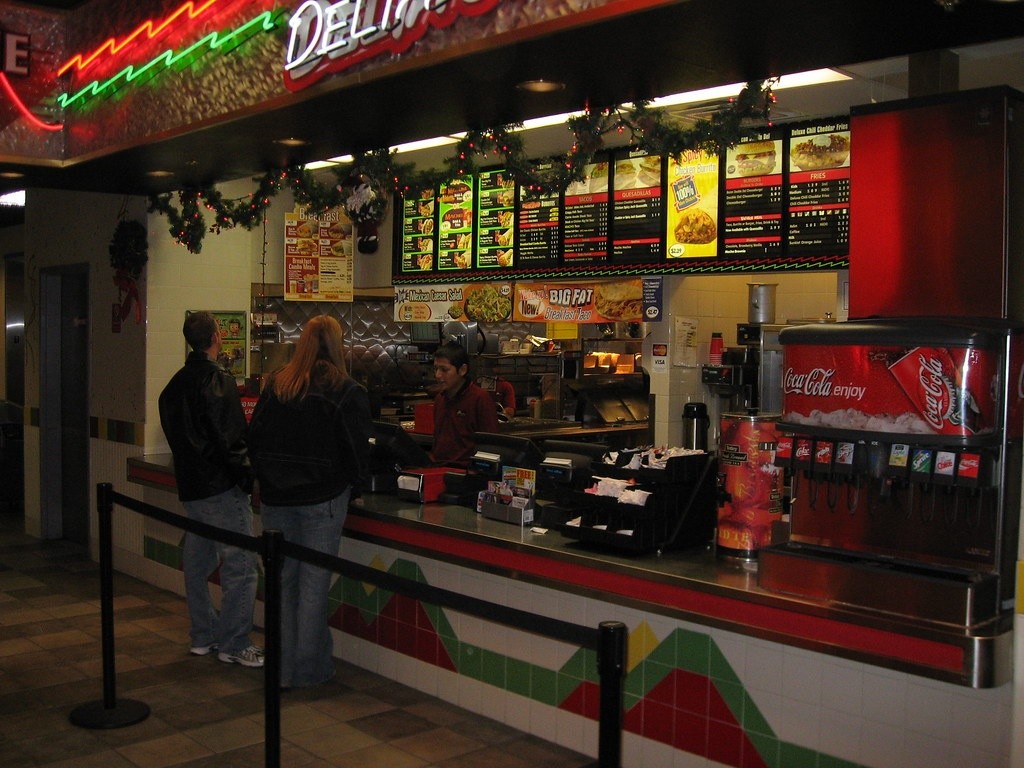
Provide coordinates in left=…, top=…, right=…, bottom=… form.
left=682, top=402, right=710, bottom=453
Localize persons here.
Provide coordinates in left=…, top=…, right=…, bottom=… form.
left=159, top=311, right=264, bottom=666
left=246, top=313, right=376, bottom=692
left=481, top=366, right=516, bottom=419
left=428, top=341, right=500, bottom=467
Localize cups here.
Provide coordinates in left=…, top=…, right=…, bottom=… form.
left=710, top=338, right=724, bottom=364
left=312, top=279, right=318, bottom=292
left=298, top=280, right=304, bottom=292
left=290, top=281, right=296, bottom=292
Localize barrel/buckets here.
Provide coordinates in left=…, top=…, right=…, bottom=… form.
left=747, top=283, right=778, bottom=323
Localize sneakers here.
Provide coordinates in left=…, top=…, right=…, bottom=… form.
left=190, top=641, right=266, bottom=667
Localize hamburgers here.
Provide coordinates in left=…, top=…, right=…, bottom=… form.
left=734, top=141, right=776, bottom=177
left=617, top=159, right=636, bottom=189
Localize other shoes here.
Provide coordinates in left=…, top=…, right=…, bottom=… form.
left=279, top=673, right=344, bottom=692
